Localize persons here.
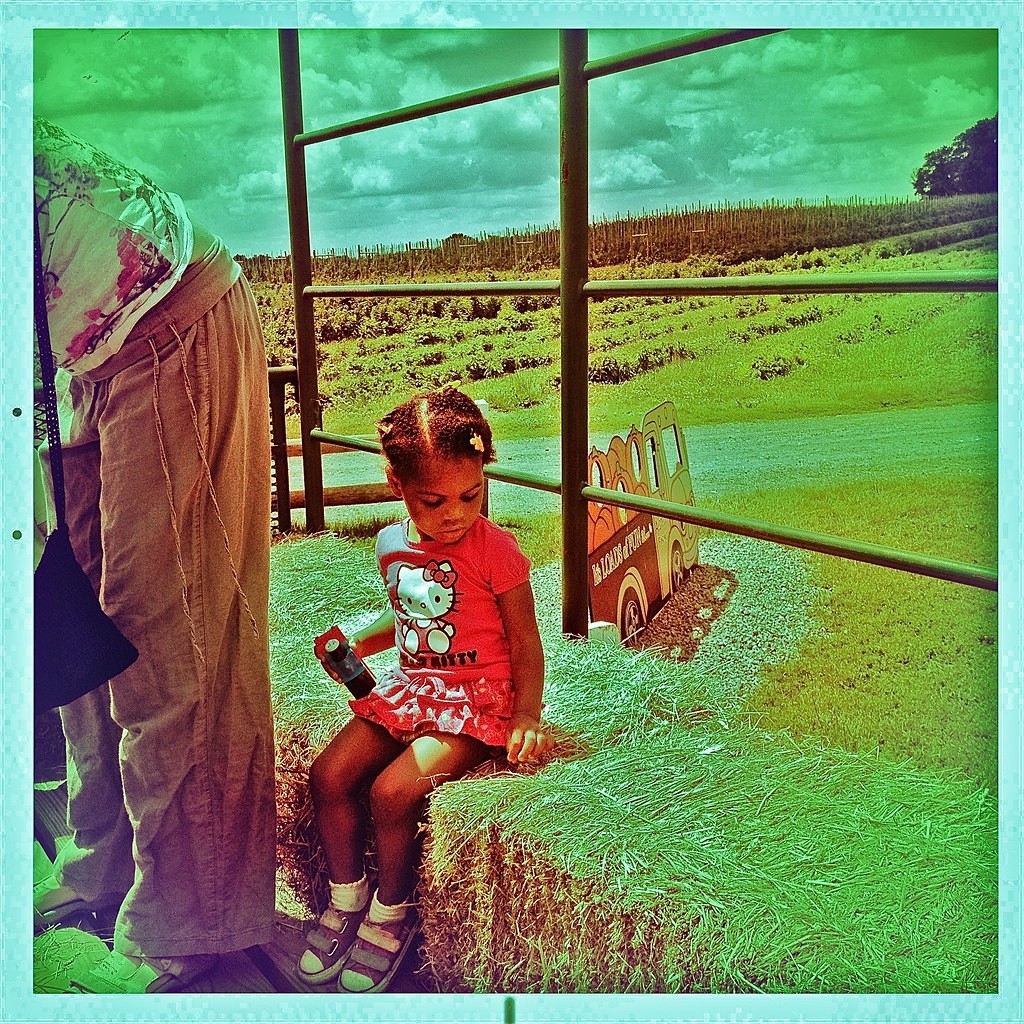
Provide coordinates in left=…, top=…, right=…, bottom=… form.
left=34, top=114, right=276, bottom=995
left=298, top=388, right=555, bottom=994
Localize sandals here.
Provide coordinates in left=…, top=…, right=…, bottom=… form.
left=63, top=946, right=222, bottom=994
left=33, top=874, right=131, bottom=935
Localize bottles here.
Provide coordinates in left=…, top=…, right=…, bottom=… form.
left=324, top=639, right=377, bottom=700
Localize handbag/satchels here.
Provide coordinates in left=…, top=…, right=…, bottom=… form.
left=34, top=528, right=140, bottom=717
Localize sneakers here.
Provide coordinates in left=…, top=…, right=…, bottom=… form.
left=297, top=888, right=373, bottom=985
left=337, top=908, right=420, bottom=993
